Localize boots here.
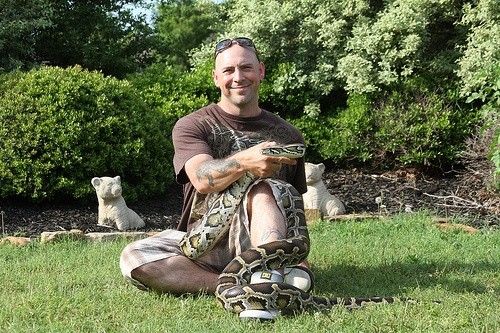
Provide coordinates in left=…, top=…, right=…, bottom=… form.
left=238, top=271, right=284, bottom=323
left=284, top=266, right=315, bottom=293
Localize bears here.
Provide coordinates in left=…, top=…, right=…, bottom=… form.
left=302, top=162, right=345, bottom=215
left=91, top=175, right=146, bottom=230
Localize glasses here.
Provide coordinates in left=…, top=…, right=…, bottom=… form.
left=215, top=37, right=260, bottom=61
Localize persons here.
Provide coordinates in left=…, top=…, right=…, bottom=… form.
left=118, top=36, right=314, bottom=323
